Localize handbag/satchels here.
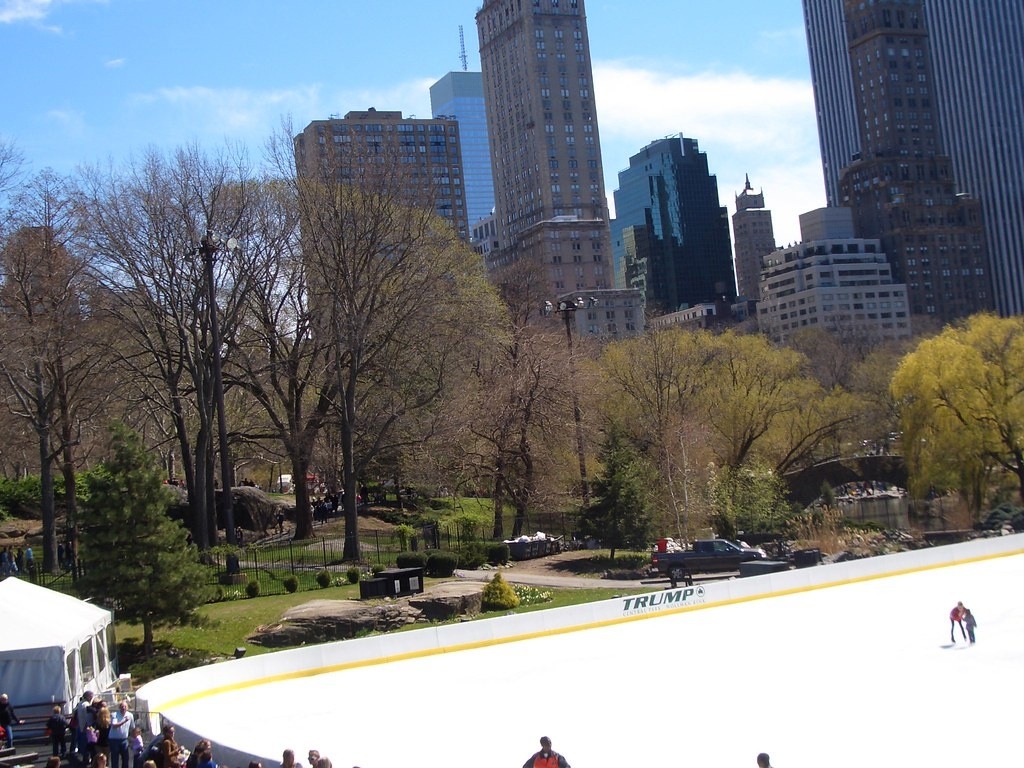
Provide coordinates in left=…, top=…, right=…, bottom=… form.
left=86, top=730, right=99, bottom=743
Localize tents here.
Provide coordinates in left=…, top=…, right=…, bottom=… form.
left=277, top=473, right=294, bottom=494
left=0, top=575, right=120, bottom=739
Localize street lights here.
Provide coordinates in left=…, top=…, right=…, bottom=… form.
left=544, top=296, right=600, bottom=509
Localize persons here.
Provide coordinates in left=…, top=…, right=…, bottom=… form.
left=163, top=478, right=184, bottom=487
left=214, top=479, right=219, bottom=489
left=394, top=484, right=448, bottom=500
left=0, top=543, right=33, bottom=575
left=58, top=542, right=71, bottom=560
left=310, top=483, right=369, bottom=525
left=949, top=602, right=977, bottom=643
left=234, top=526, right=244, bottom=544
left=847, top=444, right=906, bottom=456
left=277, top=510, right=284, bottom=533
left=0, top=694, right=25, bottom=748
left=757, top=753, right=773, bottom=768
left=837, top=480, right=888, bottom=497
left=46, top=690, right=218, bottom=768
left=522, top=736, right=571, bottom=768
left=249, top=749, right=361, bottom=768
left=242, top=477, right=255, bottom=487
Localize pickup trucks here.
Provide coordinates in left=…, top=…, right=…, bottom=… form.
left=651, top=540, right=766, bottom=582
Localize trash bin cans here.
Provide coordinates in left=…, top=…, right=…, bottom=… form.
left=794, top=548, right=820, bottom=569
left=506, top=539, right=551, bottom=561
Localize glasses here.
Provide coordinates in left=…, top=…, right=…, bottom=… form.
left=308, top=758, right=318, bottom=761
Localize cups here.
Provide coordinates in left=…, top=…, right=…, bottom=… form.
left=51, top=696, right=54, bottom=704
